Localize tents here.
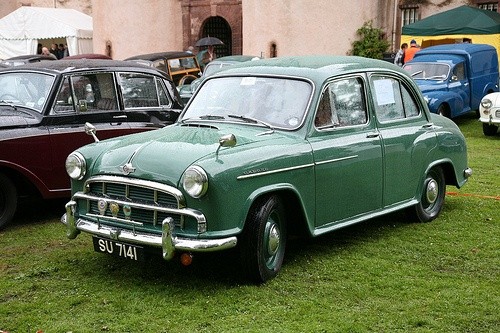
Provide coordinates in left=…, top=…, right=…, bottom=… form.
left=0, top=6, right=94, bottom=64
left=400, top=5, right=500, bottom=72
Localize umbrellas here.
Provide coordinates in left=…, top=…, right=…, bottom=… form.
left=195, top=35, right=224, bottom=61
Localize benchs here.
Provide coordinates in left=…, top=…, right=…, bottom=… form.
left=96, top=97, right=157, bottom=111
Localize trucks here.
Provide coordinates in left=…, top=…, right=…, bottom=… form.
left=398, top=43, right=500, bottom=123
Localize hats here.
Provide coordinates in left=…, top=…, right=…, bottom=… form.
left=411, top=40, right=416, bottom=46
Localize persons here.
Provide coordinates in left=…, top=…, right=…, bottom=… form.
left=185, top=46, right=194, bottom=54
left=38, top=43, right=69, bottom=59
left=203, top=46, right=216, bottom=65
left=41, top=47, right=57, bottom=60
left=394, top=43, right=408, bottom=67
left=401, top=40, right=422, bottom=66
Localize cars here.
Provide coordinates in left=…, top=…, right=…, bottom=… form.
left=0, top=58, right=184, bottom=228
left=7, top=50, right=201, bottom=106
left=479, top=92, right=500, bottom=137
left=60, top=54, right=472, bottom=282
